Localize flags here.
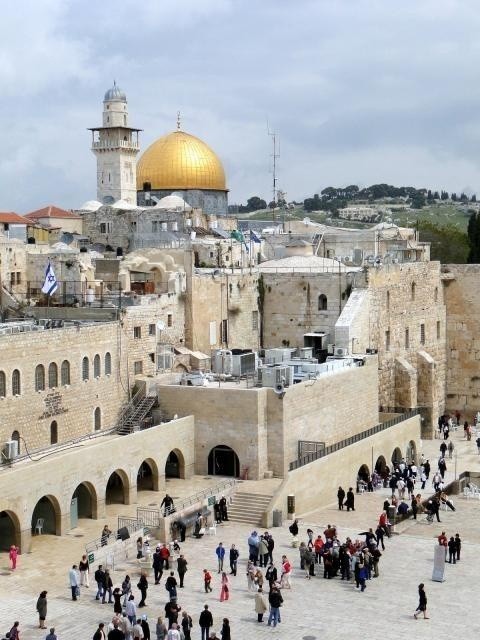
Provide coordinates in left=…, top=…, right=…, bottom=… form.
left=231, top=229, right=245, bottom=241
left=40, top=260, right=58, bottom=297
left=250, top=230, right=261, bottom=243
left=241, top=231, right=250, bottom=254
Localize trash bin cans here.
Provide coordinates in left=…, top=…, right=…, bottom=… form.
left=273, top=509, right=283, bottom=527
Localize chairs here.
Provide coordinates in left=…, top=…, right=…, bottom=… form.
left=358, top=484, right=364, bottom=494
left=209, top=521, right=217, bottom=536
left=440, top=499, right=453, bottom=511
left=199, top=528, right=205, bottom=535
left=35, top=518, right=44, bottom=535
left=378, top=479, right=384, bottom=488
left=463, top=482, right=480, bottom=501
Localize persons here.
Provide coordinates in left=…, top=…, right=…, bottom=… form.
left=1, top=493, right=461, bottom=640
left=337, top=407, right=480, bottom=515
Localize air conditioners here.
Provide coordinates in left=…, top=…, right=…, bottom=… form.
left=5, top=440, right=18, bottom=459
left=176, top=205, right=184, bottom=212
left=212, top=348, right=347, bottom=384
left=328, top=344, right=334, bottom=354
left=334, top=256, right=341, bottom=262
left=344, top=256, right=352, bottom=262
